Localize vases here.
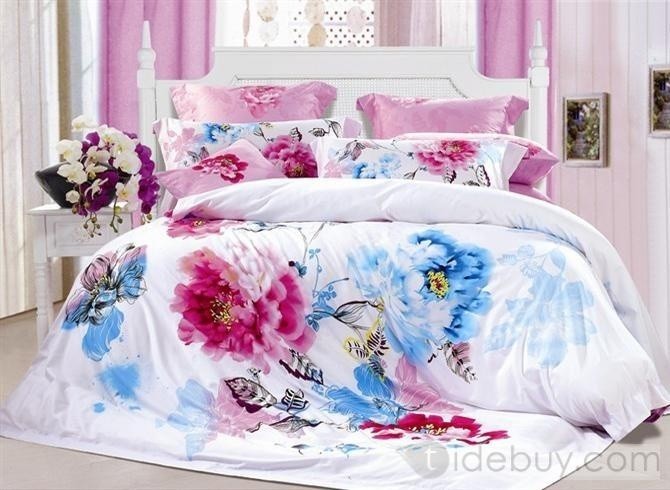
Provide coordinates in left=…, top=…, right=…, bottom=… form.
left=35, top=159, right=132, bottom=209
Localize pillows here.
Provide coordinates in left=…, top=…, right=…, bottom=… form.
left=152, top=82, right=559, bottom=216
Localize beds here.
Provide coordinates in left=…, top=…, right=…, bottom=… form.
left=1, top=20, right=670, bottom=489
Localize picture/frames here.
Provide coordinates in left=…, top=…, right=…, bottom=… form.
left=560, top=92, right=608, bottom=169
left=647, top=63, right=670, bottom=139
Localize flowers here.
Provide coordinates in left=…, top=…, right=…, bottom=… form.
left=56, top=113, right=160, bottom=238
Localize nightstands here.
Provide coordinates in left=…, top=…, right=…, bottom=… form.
left=23, top=201, right=132, bottom=356
